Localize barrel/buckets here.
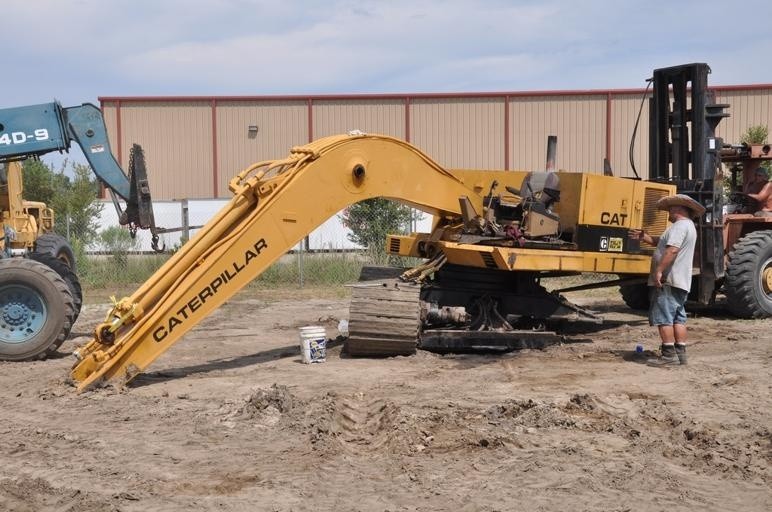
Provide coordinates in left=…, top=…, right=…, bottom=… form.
left=299, top=326, right=327, bottom=364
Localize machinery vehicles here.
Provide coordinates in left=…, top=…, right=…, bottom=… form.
left=0, top=98, right=166, bottom=362
left=1, top=157, right=76, bottom=272
left=69, top=61, right=772, bottom=393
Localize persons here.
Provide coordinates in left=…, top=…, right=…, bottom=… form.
left=735, top=168, right=772, bottom=211
left=627, top=194, right=706, bottom=367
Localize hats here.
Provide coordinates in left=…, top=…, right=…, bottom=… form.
left=655, top=194, right=706, bottom=219
left=752, top=167, right=768, bottom=175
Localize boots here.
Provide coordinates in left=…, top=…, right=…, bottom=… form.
left=674, top=343, right=689, bottom=365
left=646, top=343, right=681, bottom=370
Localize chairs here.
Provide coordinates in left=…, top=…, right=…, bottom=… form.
left=498, top=168, right=561, bottom=223
